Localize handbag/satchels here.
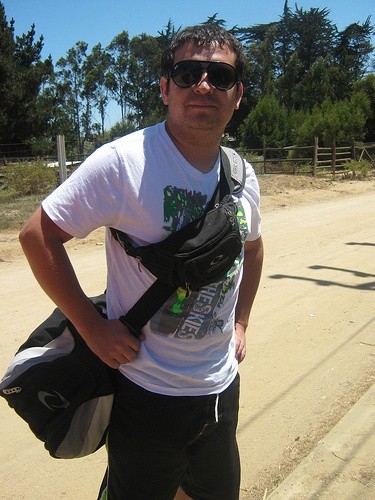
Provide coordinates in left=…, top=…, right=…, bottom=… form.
left=1, top=290, right=116, bottom=460
left=124, top=194, right=242, bottom=294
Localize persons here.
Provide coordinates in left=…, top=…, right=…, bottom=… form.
left=18, top=25, right=264, bottom=500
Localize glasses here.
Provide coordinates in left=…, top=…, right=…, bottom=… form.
left=164, top=59, right=241, bottom=92
